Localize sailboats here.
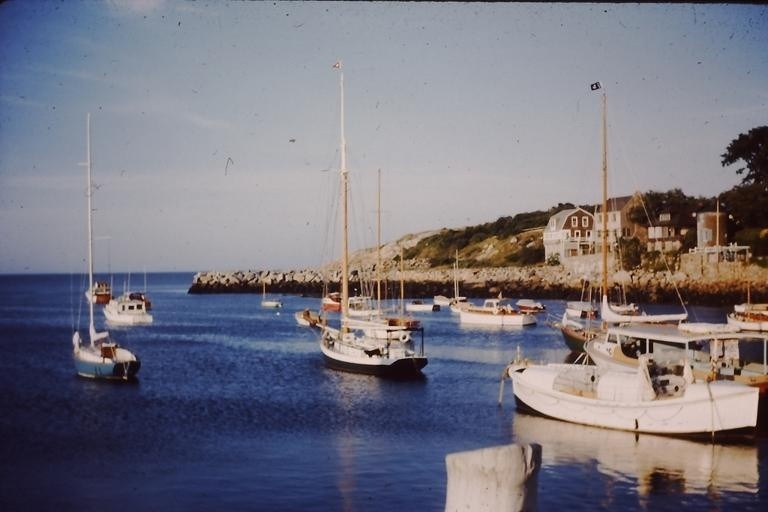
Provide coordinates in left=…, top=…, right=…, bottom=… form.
left=320, top=172, right=429, bottom=377
left=560, top=82, right=705, bottom=357
left=257, top=266, right=283, bottom=310
left=291, top=168, right=421, bottom=335
left=433, top=249, right=463, bottom=309
left=72, top=112, right=140, bottom=382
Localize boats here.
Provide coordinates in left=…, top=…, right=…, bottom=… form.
left=120, top=269, right=151, bottom=311
left=508, top=344, right=760, bottom=433
left=514, top=298, right=543, bottom=316
left=102, top=271, right=153, bottom=326
left=609, top=302, right=638, bottom=313
left=403, top=295, right=442, bottom=313
left=584, top=324, right=768, bottom=394
left=726, top=304, right=768, bottom=332
left=85, top=280, right=113, bottom=305
left=459, top=287, right=538, bottom=327
left=565, top=301, right=598, bottom=320
left=451, top=262, right=512, bottom=315
left=511, top=407, right=761, bottom=494
